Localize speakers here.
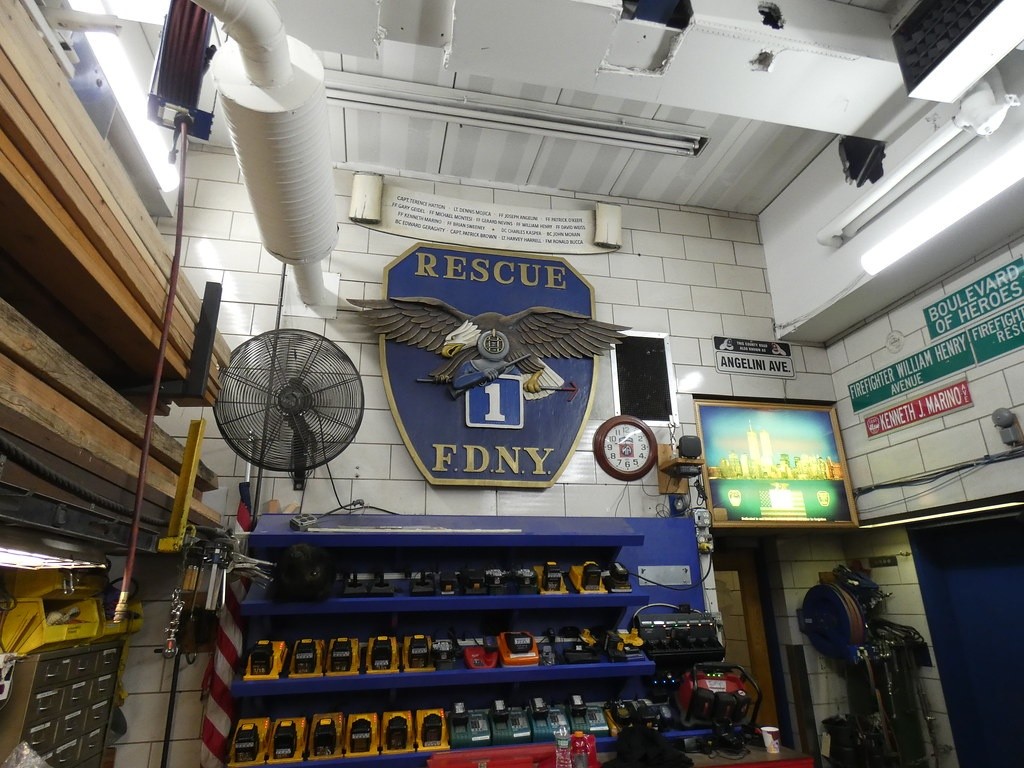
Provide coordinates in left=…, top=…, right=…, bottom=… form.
left=679, top=436, right=701, bottom=457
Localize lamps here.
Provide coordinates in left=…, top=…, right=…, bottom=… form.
left=817, top=115, right=982, bottom=249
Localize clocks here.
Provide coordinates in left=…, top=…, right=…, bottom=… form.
left=594, top=414, right=658, bottom=481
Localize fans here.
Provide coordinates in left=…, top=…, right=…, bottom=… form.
left=215, top=328, right=364, bottom=491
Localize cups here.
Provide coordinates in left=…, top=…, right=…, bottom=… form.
left=761, top=727, right=779, bottom=753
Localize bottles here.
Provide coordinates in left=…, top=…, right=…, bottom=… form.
left=573, top=731, right=588, bottom=768
left=554, top=721, right=572, bottom=768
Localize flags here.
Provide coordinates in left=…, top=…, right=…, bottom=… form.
left=200, top=482, right=251, bottom=768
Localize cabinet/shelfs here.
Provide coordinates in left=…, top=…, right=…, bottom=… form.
left=227, top=515, right=714, bottom=768
left=0, top=639, right=126, bottom=768
